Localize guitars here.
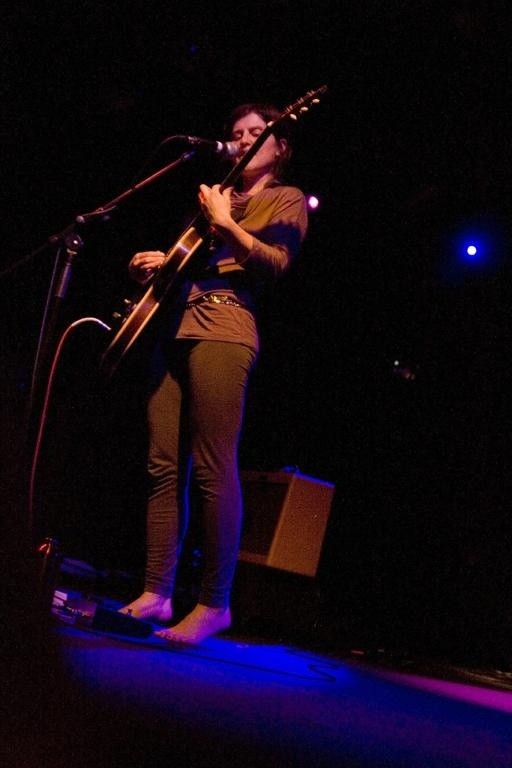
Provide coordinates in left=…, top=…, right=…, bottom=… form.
left=99, top=86, right=328, bottom=369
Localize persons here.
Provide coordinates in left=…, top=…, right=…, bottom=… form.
left=97, top=95, right=310, bottom=644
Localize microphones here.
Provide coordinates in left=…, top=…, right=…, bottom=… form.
left=189, top=131, right=241, bottom=162
